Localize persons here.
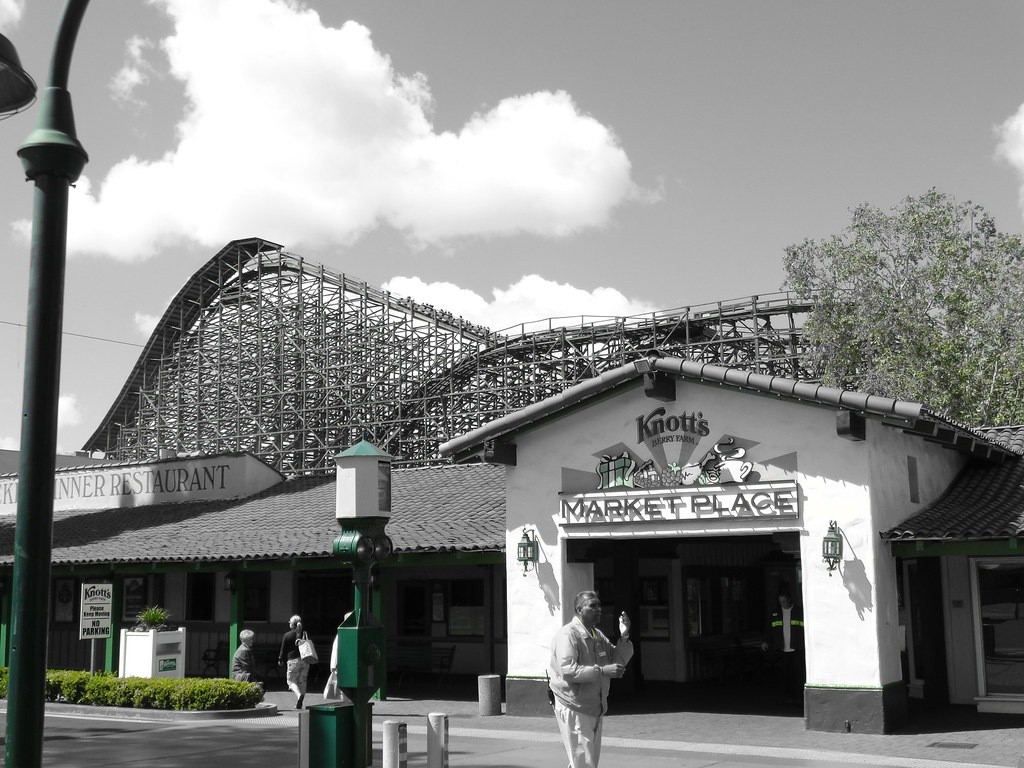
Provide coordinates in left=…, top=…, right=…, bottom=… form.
left=231, top=630, right=260, bottom=682
left=278, top=615, right=308, bottom=709
left=547, top=591, right=634, bottom=768
left=760, top=590, right=804, bottom=705
left=329, top=610, right=353, bottom=672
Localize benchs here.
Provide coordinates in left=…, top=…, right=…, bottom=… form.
left=201, top=640, right=336, bottom=686
left=387, top=643, right=456, bottom=687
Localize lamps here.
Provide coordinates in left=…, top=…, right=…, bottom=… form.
left=821, top=520, right=845, bottom=576
left=515, top=525, right=538, bottom=578
left=224, top=571, right=239, bottom=595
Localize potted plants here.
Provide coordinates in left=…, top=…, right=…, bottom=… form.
left=139, top=607, right=173, bottom=629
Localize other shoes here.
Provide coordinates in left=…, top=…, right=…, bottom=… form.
left=296, top=694, right=304, bottom=709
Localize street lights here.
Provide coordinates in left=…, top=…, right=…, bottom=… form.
left=331, top=439, right=394, bottom=768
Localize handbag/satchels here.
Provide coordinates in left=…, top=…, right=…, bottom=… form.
left=295, top=630, right=318, bottom=662
left=324, top=668, right=340, bottom=700
